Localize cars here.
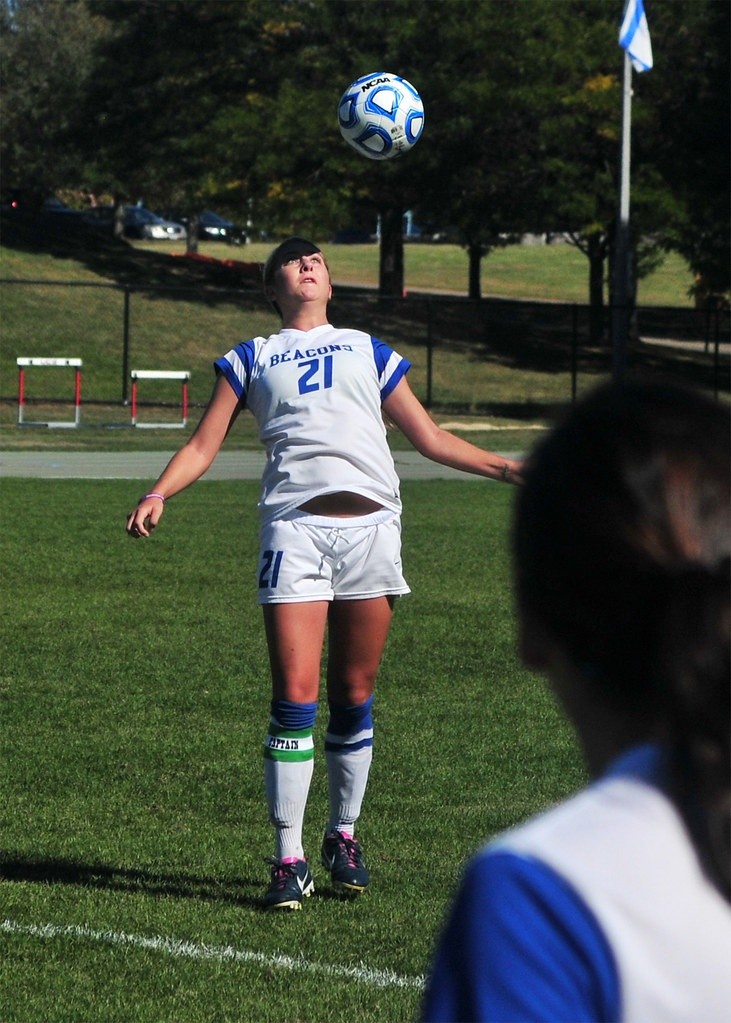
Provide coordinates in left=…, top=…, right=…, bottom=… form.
left=7, top=185, right=82, bottom=221
left=155, top=205, right=243, bottom=244
left=84, top=204, right=186, bottom=242
left=369, top=222, right=437, bottom=246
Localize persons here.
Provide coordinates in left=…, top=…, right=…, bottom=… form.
left=126, top=237, right=523, bottom=910
left=420, top=374, right=731, bottom=1023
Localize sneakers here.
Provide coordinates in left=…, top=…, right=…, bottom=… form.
left=321, top=829, right=369, bottom=889
left=262, top=856, right=314, bottom=910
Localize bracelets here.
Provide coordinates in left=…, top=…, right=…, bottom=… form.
left=137, top=493, right=164, bottom=503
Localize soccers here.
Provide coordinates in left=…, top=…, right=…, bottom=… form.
left=336, top=71, right=426, bottom=161
